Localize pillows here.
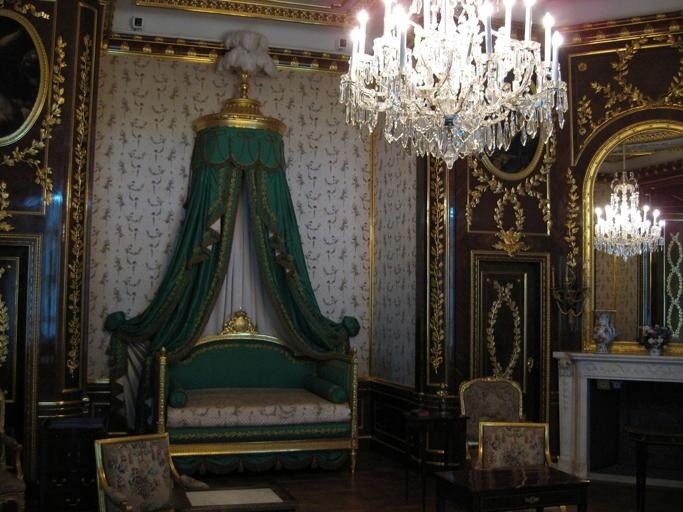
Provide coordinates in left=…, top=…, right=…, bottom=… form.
left=303, top=376, right=347, bottom=403
left=170, top=383, right=187, bottom=408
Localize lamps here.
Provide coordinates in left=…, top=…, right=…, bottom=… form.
left=593, top=143, right=669, bottom=263
left=338, top=0, right=569, bottom=170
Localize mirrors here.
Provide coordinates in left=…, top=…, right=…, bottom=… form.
left=580, top=118, right=683, bottom=354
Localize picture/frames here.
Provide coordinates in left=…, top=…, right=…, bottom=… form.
left=480, top=77, right=546, bottom=182
left=0, top=231, right=44, bottom=481
left=468, top=248, right=552, bottom=423
left=0, top=8, right=50, bottom=147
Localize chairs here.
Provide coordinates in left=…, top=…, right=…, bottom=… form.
left=90, top=431, right=210, bottom=512
left=135, top=310, right=360, bottom=475
left=459, top=376, right=523, bottom=461
left=475, top=420, right=568, bottom=511
left=0, top=390, right=26, bottom=512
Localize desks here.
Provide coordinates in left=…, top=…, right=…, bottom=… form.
left=433, top=463, right=590, bottom=512
left=172, top=485, right=295, bottom=511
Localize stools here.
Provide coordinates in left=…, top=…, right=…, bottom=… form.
left=629, top=424, right=682, bottom=512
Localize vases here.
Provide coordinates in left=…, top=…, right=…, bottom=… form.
left=649, top=344, right=662, bottom=356
left=636, top=324, right=671, bottom=348
left=591, top=309, right=617, bottom=354
